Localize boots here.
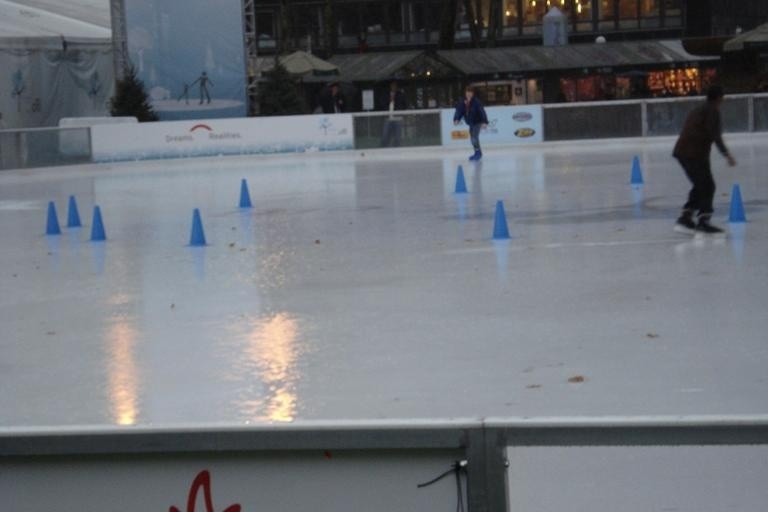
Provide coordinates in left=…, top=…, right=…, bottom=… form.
left=676, top=208, right=696, bottom=229
left=696, top=209, right=724, bottom=234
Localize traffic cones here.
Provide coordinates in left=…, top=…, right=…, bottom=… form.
left=237, top=176, right=254, bottom=209
left=724, top=182, right=751, bottom=224
left=184, top=207, right=209, bottom=247
left=489, top=198, right=514, bottom=242
left=89, top=204, right=107, bottom=241
left=65, top=195, right=83, bottom=228
left=450, top=163, right=468, bottom=195
left=43, top=199, right=63, bottom=236
left=628, top=153, right=644, bottom=186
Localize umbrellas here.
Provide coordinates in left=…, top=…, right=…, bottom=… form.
left=722, top=21, right=767, bottom=53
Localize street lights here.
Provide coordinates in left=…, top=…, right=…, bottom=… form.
left=593, top=35, right=608, bottom=99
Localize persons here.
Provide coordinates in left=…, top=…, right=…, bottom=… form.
left=311, top=84, right=345, bottom=114
left=177, top=77, right=192, bottom=105
left=377, top=78, right=410, bottom=147
left=191, top=68, right=219, bottom=106
left=671, top=84, right=736, bottom=238
left=452, top=84, right=490, bottom=162
